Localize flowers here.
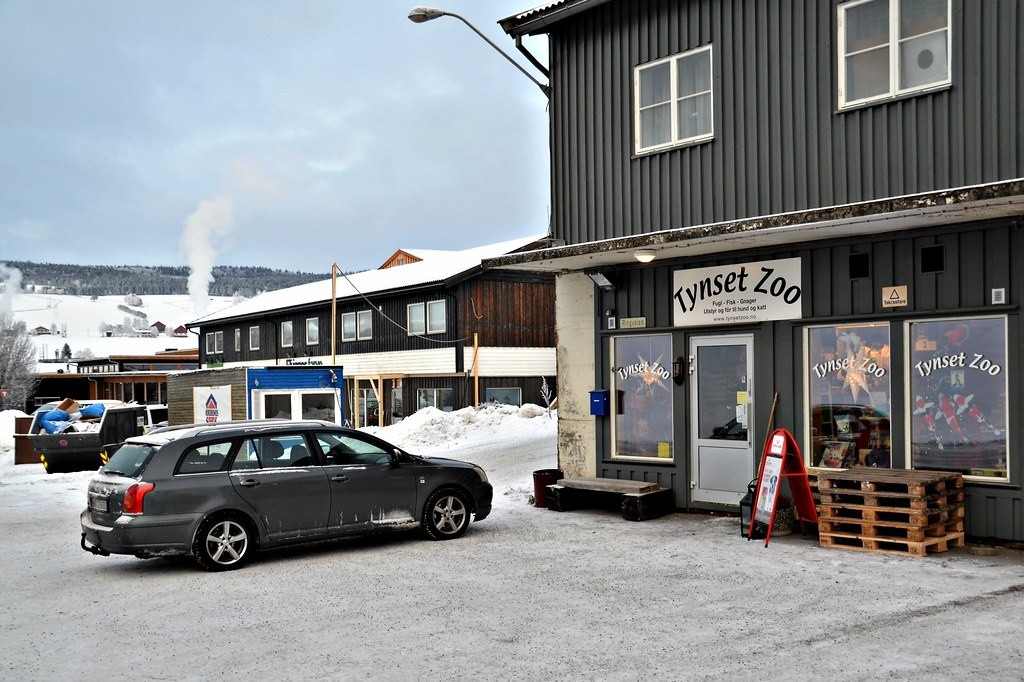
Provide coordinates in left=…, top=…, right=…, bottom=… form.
left=775, top=494, right=792, bottom=511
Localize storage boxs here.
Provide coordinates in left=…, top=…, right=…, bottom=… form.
left=56, top=398, right=80, bottom=414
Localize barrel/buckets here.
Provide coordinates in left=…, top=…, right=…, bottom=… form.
left=760, top=496, right=792, bottom=536
left=533, top=469, right=564, bottom=507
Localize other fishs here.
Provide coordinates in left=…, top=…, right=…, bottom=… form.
left=936, top=393, right=970, bottom=445
left=953, top=394, right=1002, bottom=437
left=912, top=395, right=945, bottom=451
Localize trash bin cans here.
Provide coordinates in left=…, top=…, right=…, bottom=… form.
left=533, top=468, right=564, bottom=507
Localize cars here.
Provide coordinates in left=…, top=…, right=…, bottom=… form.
left=78, top=416, right=494, bottom=574
left=708, top=405, right=891, bottom=467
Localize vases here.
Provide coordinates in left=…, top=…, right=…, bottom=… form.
left=759, top=508, right=793, bottom=536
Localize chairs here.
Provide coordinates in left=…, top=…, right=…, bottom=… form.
left=289, top=444, right=310, bottom=468
left=266, top=441, right=285, bottom=467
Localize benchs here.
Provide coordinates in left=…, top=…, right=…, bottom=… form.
left=546, top=477, right=675, bottom=522
left=178, top=450, right=226, bottom=473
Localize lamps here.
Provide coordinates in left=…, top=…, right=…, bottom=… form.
left=286, top=348, right=292, bottom=358
left=408, top=6, right=550, bottom=99
left=634, top=250, right=656, bottom=262
left=303, top=346, right=310, bottom=357
left=672, top=356, right=685, bottom=386
left=583, top=269, right=618, bottom=293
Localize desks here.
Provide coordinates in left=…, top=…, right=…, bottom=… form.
left=858, top=417, right=888, bottom=450
